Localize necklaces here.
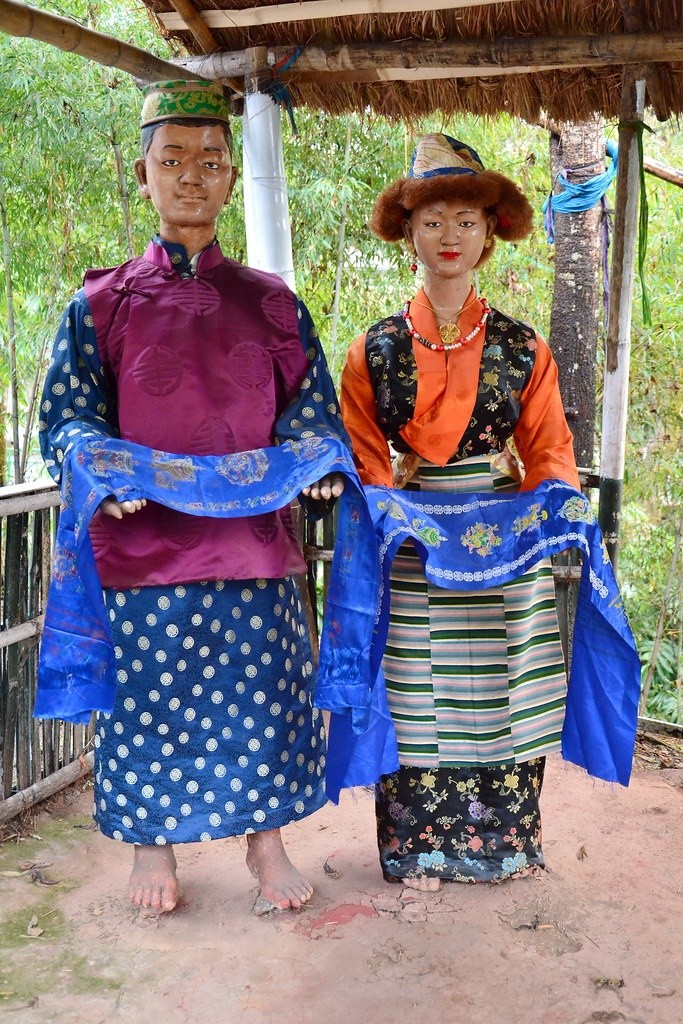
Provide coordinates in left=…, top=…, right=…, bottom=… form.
left=409, top=297, right=479, bottom=344
left=404, top=294, right=491, bottom=352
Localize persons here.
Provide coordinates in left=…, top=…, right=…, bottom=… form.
left=36, top=80, right=347, bottom=913
left=340, top=131, right=588, bottom=893
